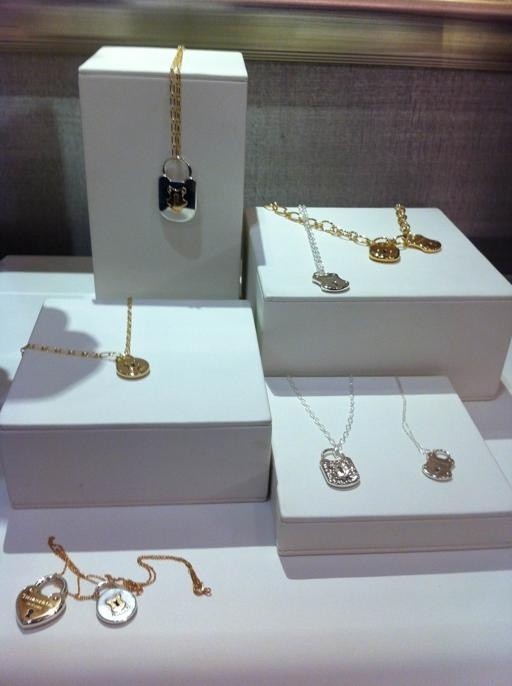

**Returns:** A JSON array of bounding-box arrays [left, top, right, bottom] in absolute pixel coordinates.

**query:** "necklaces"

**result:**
[[157, 42, 198, 225], [286, 372, 359, 489], [263, 194, 444, 292], [20, 295, 149, 380], [395, 376, 456, 483], [13, 535, 211, 631]]
